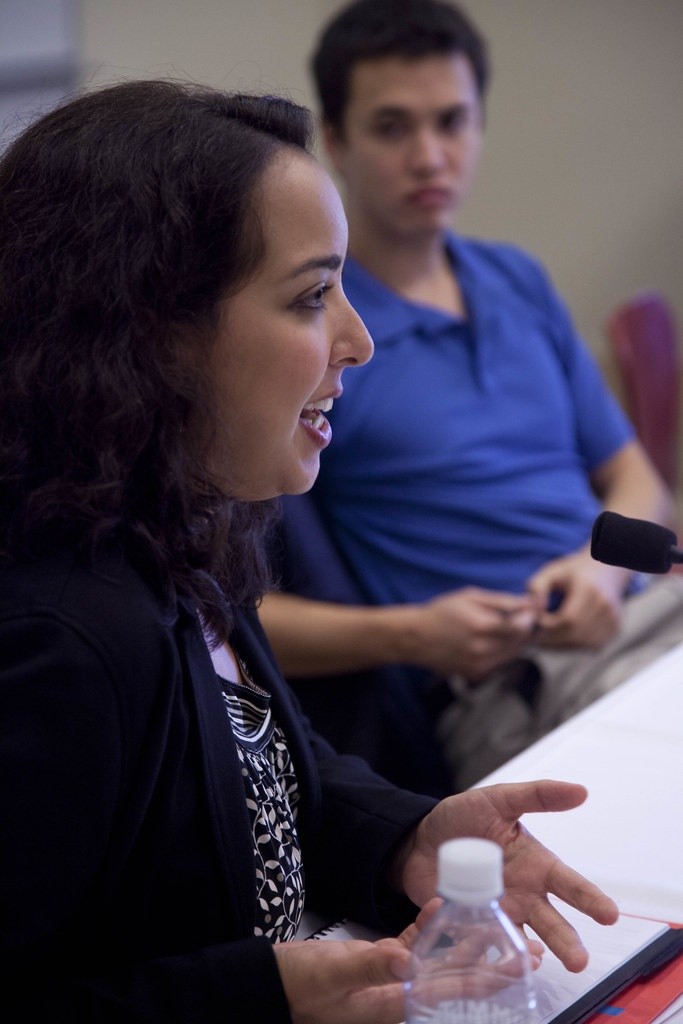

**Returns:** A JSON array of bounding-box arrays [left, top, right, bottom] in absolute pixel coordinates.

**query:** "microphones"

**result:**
[[590, 511, 683, 575]]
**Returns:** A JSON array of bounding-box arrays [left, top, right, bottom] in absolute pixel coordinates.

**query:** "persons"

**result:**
[[2, 82, 620, 1024], [254, 0, 681, 788]]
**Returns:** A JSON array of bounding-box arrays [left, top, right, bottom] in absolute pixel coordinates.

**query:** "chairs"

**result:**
[[609, 292, 683, 495]]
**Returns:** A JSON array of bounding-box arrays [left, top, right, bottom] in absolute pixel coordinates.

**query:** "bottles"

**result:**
[[405, 837, 538, 1024]]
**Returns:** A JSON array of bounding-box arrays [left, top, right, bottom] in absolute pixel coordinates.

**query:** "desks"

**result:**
[[284, 643, 683, 1024]]
